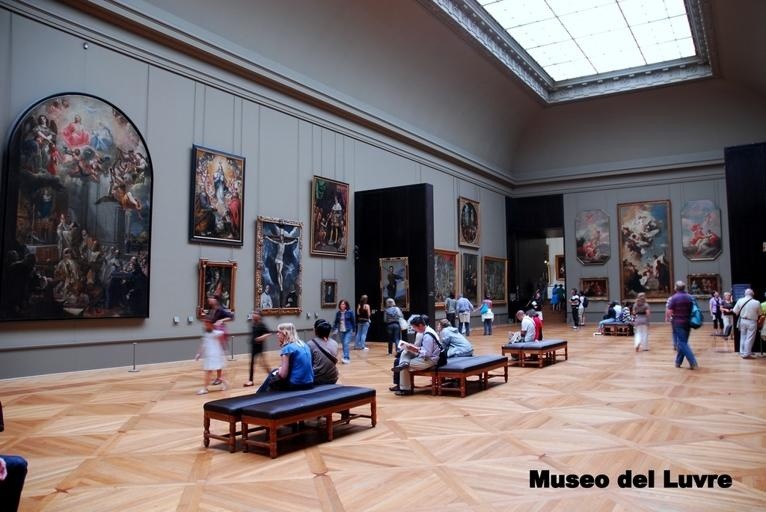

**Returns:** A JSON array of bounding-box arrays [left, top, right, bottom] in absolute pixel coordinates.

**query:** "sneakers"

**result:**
[[362, 347, 369, 350], [573, 326, 578, 329], [197, 390, 209, 396], [354, 347, 361, 350], [594, 332, 601, 334], [213, 378, 223, 385], [483, 333, 485, 335], [488, 333, 492, 335], [466, 333, 469, 335], [342, 359, 351, 364]]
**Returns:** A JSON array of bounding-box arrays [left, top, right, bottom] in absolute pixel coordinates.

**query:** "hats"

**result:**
[[387, 298, 396, 307], [607, 301, 613, 304]]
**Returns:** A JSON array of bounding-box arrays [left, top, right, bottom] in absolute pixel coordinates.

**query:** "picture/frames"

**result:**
[[311, 175, 349, 257], [0, 92, 154, 323], [544, 264, 551, 285], [555, 255, 565, 281], [196, 257, 238, 323], [579, 278, 609, 302], [481, 255, 508, 306], [434, 248, 459, 309], [616, 199, 675, 304], [458, 196, 481, 248], [574, 209, 611, 266], [188, 144, 246, 246], [687, 273, 721, 300], [462, 253, 478, 308], [321, 279, 338, 308], [545, 245, 549, 262], [379, 257, 410, 312], [254, 215, 304, 316], [680, 200, 723, 262]]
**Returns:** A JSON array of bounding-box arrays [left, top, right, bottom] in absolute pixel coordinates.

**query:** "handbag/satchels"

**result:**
[[399, 317, 408, 331], [480, 302, 489, 316], [311, 337, 340, 367], [421, 331, 448, 367], [603, 314, 611, 318], [691, 292, 705, 328], [737, 298, 751, 326]]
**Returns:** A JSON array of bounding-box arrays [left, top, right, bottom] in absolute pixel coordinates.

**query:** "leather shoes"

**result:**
[[395, 390, 412, 396], [691, 366, 698, 368], [391, 361, 410, 372], [243, 383, 254, 387], [676, 363, 681, 367], [390, 385, 400, 392]]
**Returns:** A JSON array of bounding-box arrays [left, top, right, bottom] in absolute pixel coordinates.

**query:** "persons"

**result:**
[[568, 288, 581, 329], [208, 272, 230, 310], [577, 290, 586, 325], [21, 100, 148, 220], [555, 284, 565, 310], [0, 401, 28, 511], [264, 232, 298, 291], [624, 255, 668, 293], [306, 319, 339, 385], [261, 284, 273, 308], [385, 265, 403, 298], [475, 296, 494, 336], [508, 310, 536, 361], [720, 292, 734, 340], [614, 300, 622, 321], [285, 285, 299, 307], [526, 309, 543, 343], [621, 301, 634, 326], [455, 292, 474, 336], [632, 292, 650, 352], [388, 315, 439, 392], [196, 184, 220, 238], [732, 288, 761, 360], [445, 290, 457, 328], [195, 322, 228, 395], [326, 287, 334, 302], [436, 318, 474, 358], [333, 299, 356, 364], [527, 292, 543, 321], [709, 290, 724, 335], [329, 195, 344, 244], [391, 317, 443, 396], [550, 284, 559, 313], [668, 281, 700, 370], [212, 162, 229, 208], [315, 206, 329, 248], [384, 298, 404, 355], [354, 295, 371, 350], [223, 186, 241, 238], [594, 303, 616, 335], [665, 293, 678, 350], [243, 310, 274, 387], [9, 215, 147, 316], [204, 298, 234, 385], [255, 323, 313, 392]]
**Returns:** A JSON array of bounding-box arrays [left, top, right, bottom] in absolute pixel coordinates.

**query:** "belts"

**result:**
[[387, 321, 399, 325], [635, 311, 646, 313]]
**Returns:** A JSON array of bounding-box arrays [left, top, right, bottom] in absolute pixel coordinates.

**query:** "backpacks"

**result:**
[[583, 297, 588, 308], [692, 298, 704, 329]]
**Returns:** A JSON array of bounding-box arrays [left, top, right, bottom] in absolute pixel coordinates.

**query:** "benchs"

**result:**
[[203, 383, 344, 453], [603, 321, 622, 336], [437, 355, 508, 398], [522, 339, 567, 368], [617, 322, 634, 336], [240, 386, 376, 458], [501, 339, 546, 367], [410, 354, 481, 396]]
